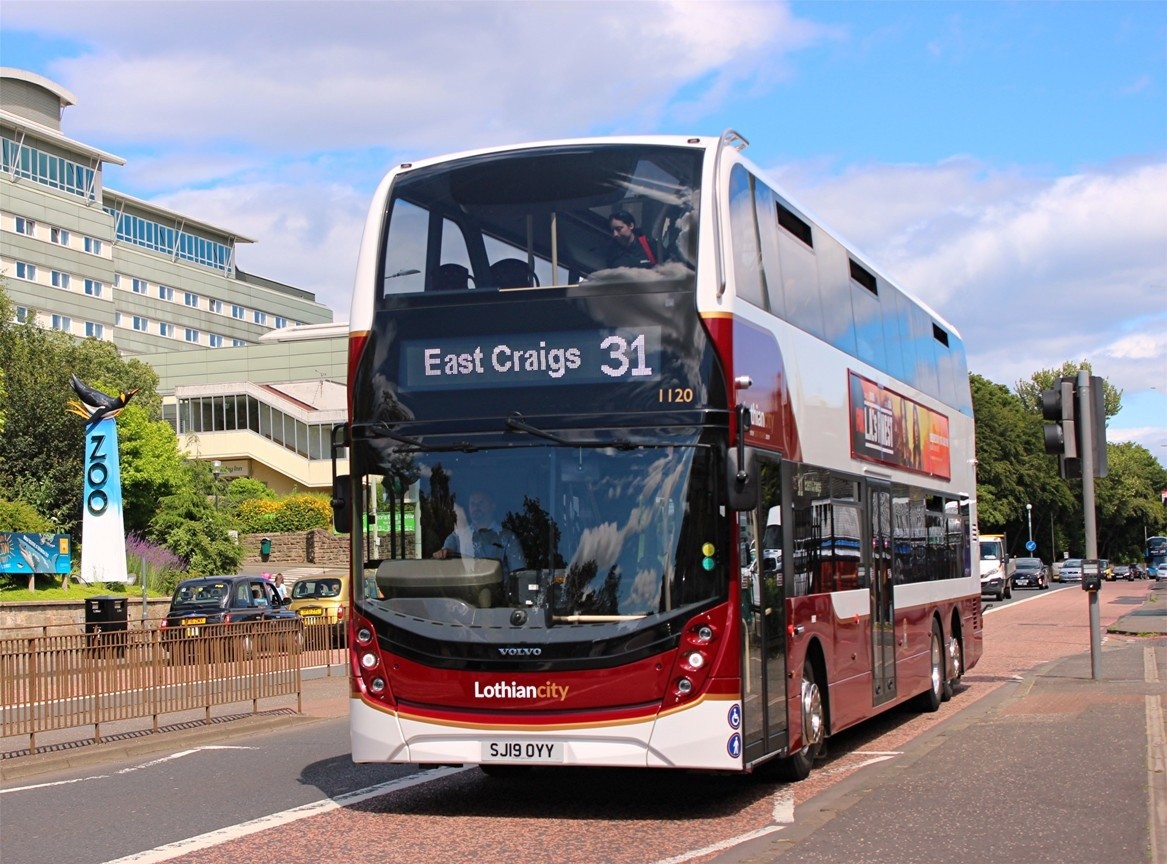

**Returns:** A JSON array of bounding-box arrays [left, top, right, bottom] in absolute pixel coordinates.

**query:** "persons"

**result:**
[[331, 582, 340, 596], [432, 485, 528, 608], [267, 572, 289, 611], [608, 210, 669, 270], [251, 589, 268, 606]]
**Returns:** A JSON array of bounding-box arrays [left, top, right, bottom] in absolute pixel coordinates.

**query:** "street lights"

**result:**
[[1026, 504, 1033, 558]]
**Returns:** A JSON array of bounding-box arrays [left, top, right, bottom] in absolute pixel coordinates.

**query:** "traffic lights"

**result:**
[[1041, 376, 1082, 480]]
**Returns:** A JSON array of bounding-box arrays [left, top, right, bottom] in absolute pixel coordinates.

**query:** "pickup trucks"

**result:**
[[978, 532, 1017, 602]]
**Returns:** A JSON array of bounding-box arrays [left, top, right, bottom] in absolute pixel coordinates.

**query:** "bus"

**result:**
[[1142, 536, 1167, 580], [330, 127, 986, 784]]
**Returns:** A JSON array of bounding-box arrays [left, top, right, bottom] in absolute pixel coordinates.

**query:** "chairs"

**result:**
[[982, 547, 992, 556], [895, 543, 955, 585], [181, 589, 221, 601], [764, 524, 798, 552], [431, 258, 541, 291], [252, 590, 261, 599]]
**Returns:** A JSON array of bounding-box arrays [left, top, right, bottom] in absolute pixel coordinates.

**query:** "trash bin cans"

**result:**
[[261, 538, 271, 554], [84, 595, 128, 658]]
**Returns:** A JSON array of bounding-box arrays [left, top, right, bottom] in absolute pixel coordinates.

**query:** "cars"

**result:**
[[1099, 559, 1145, 579], [1012, 557, 1050, 590], [1058, 558, 1084, 584], [1155, 563, 1167, 581], [1110, 565, 1135, 582], [268, 566, 384, 649], [161, 575, 298, 662]]
